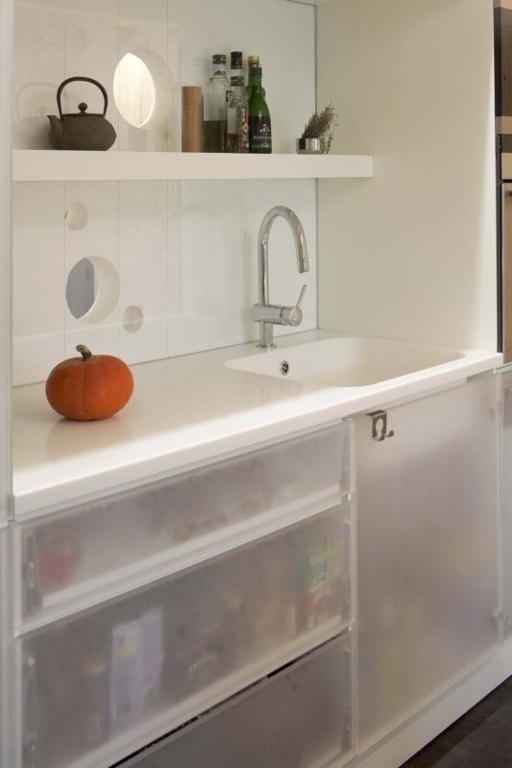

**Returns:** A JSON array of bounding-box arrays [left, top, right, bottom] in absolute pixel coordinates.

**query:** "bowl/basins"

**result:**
[[295, 137, 327, 156]]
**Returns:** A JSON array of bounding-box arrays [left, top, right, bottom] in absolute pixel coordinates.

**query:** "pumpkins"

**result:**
[[45, 345, 134, 420]]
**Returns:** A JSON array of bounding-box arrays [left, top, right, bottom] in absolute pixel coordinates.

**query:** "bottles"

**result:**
[[182, 50, 273, 151]]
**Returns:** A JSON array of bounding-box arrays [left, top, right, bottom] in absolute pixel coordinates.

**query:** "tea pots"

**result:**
[[48, 76, 116, 150]]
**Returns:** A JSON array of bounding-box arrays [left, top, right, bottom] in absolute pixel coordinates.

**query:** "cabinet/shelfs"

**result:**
[[351, 370, 503, 753], [7, 419, 353, 768]]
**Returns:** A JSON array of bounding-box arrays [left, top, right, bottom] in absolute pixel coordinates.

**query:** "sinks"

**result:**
[[222, 335, 470, 388]]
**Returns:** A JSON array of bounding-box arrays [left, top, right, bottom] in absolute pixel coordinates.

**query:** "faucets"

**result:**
[[251, 206, 311, 349]]
